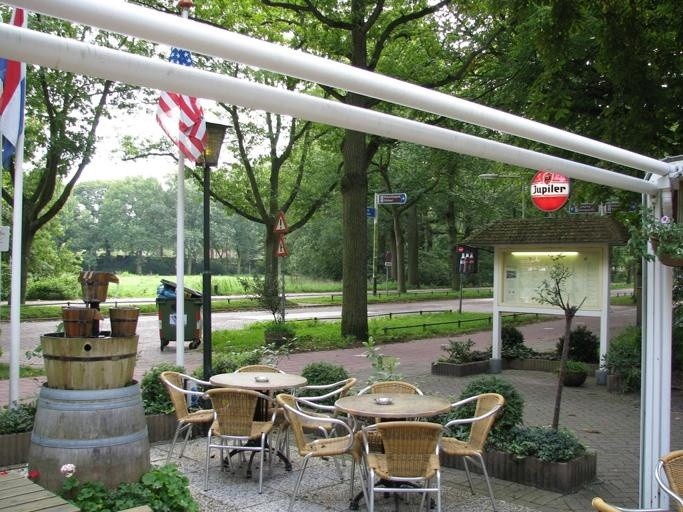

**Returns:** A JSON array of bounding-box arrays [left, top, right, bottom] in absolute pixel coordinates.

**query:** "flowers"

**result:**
[[612, 205, 683, 258]]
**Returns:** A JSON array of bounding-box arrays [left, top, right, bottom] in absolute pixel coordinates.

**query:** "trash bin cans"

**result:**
[[155, 279, 204, 351]]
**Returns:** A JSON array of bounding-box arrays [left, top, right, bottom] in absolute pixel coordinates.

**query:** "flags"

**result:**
[[155, 2, 208, 161], [1, 6, 29, 164]]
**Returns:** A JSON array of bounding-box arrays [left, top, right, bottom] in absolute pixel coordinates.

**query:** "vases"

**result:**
[[650, 235, 682, 268]]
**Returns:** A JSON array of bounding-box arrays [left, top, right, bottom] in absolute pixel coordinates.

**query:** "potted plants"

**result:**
[[237, 273, 295, 348], [556, 362, 588, 387]]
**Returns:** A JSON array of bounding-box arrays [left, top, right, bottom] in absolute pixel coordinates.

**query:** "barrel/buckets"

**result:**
[[78, 270, 119, 305], [38, 330, 140, 392], [60, 302, 95, 338], [27, 379, 152, 500], [108, 301, 139, 337]]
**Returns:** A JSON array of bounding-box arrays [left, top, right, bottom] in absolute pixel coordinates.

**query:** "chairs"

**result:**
[[358, 380, 425, 450], [233, 364, 289, 460], [276, 376, 359, 470], [162, 371, 226, 473], [354, 420, 443, 512], [656, 449, 683, 512], [277, 393, 370, 511], [333, 393, 452, 511], [438, 392, 504, 512]]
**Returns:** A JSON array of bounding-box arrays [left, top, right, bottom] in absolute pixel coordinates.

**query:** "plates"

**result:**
[[373, 398, 392, 405], [254, 376, 269, 382]]
